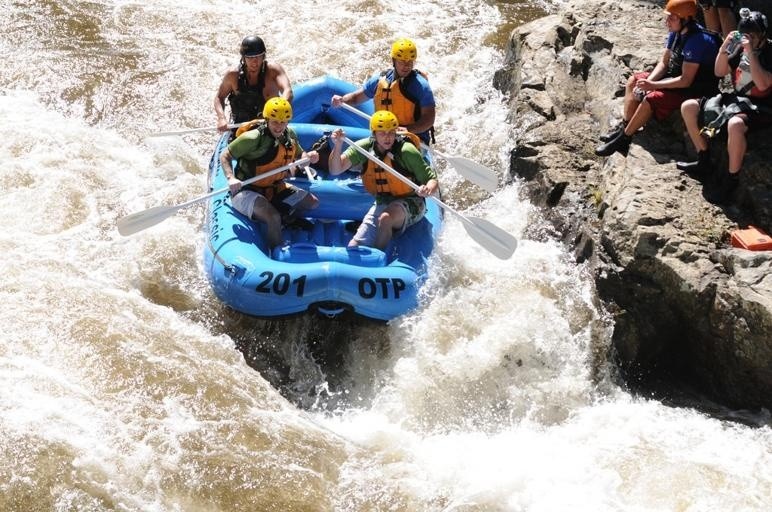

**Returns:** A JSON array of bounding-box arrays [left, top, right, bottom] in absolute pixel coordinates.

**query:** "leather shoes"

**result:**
[[709, 173, 740, 201], [600, 119, 628, 142], [596, 128, 632, 156], [676, 149, 707, 173]]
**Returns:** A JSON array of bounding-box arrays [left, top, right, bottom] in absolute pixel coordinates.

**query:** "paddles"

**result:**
[[334, 128, 517, 260], [143, 121, 251, 147], [117, 156, 311, 236], [339, 101, 498, 192]]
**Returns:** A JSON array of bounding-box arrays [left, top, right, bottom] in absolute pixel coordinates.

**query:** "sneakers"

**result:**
[[289, 218, 315, 230], [346, 220, 362, 233]]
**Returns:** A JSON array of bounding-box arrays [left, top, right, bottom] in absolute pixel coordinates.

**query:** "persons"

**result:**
[[214, 36, 293, 145], [329, 111, 439, 251], [220, 97, 319, 251], [330, 41, 436, 148], [595, 0, 723, 155], [699, 0, 736, 40], [677, 8, 772, 200]]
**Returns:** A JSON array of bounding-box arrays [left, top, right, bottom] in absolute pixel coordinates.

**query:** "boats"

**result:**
[[206, 75, 444, 320]]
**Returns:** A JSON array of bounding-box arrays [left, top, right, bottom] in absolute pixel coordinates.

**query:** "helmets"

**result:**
[[664, 1, 696, 32], [737, 7, 768, 51], [390, 38, 417, 78], [263, 97, 292, 120], [369, 110, 399, 131], [240, 34, 266, 58]]
